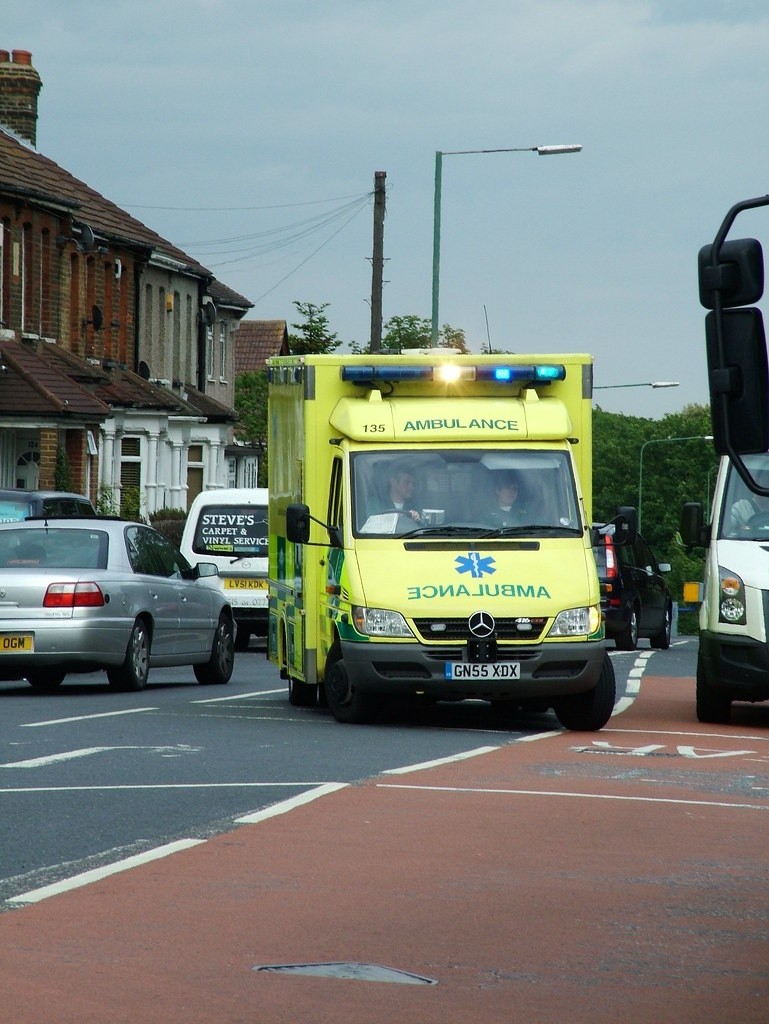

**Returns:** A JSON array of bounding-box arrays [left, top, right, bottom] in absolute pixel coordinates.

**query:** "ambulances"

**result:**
[[266, 343, 616, 732]]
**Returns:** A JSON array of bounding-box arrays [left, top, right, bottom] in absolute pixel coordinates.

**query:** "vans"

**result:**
[[177, 487, 268, 653], [1, 487, 103, 568]]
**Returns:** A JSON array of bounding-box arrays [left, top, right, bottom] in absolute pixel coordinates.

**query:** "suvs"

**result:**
[[590, 522, 672, 652]]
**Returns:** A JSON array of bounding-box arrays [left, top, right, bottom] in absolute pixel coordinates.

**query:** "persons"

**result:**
[[729, 471, 769, 526], [363, 465, 426, 527], [473, 477, 537, 529]]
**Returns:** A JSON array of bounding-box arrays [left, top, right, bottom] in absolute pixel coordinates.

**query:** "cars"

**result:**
[[0, 514, 238, 694]]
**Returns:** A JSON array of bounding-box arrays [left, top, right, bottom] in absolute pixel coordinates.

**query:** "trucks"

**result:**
[[682, 451, 769, 726]]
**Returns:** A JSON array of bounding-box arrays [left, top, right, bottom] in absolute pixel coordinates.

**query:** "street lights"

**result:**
[[637, 435, 713, 530], [429, 144, 583, 345]]
